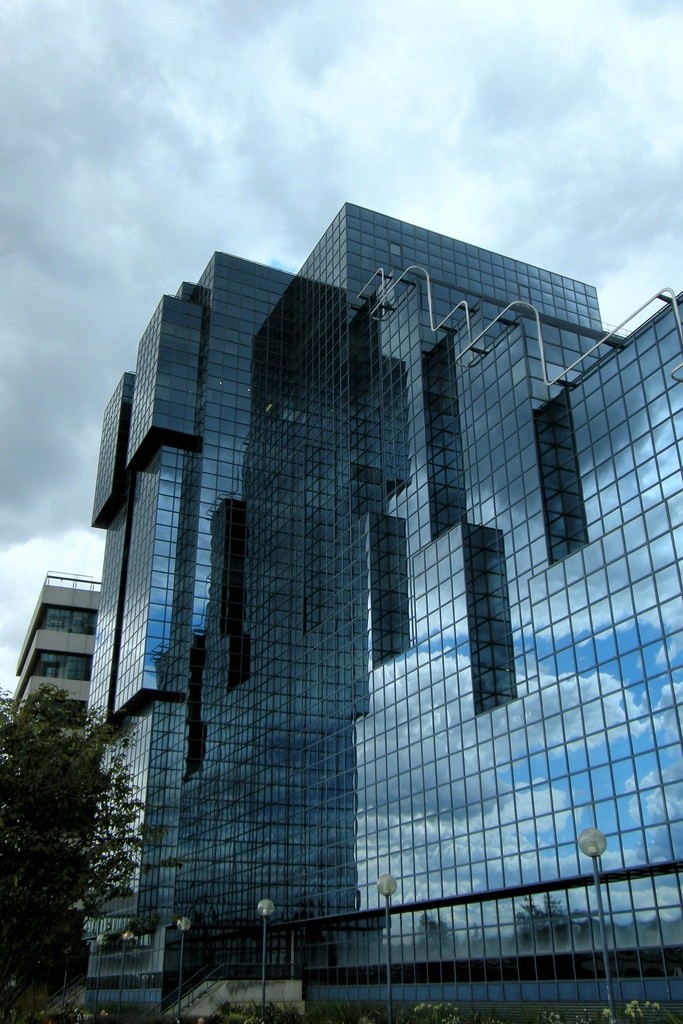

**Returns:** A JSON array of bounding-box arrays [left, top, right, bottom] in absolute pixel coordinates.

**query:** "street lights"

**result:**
[[116, 932, 135, 1024], [256, 898, 275, 1022], [377, 874, 398, 1024], [578, 828, 620, 1024], [176, 917, 192, 1024], [92, 934, 108, 1024]]
